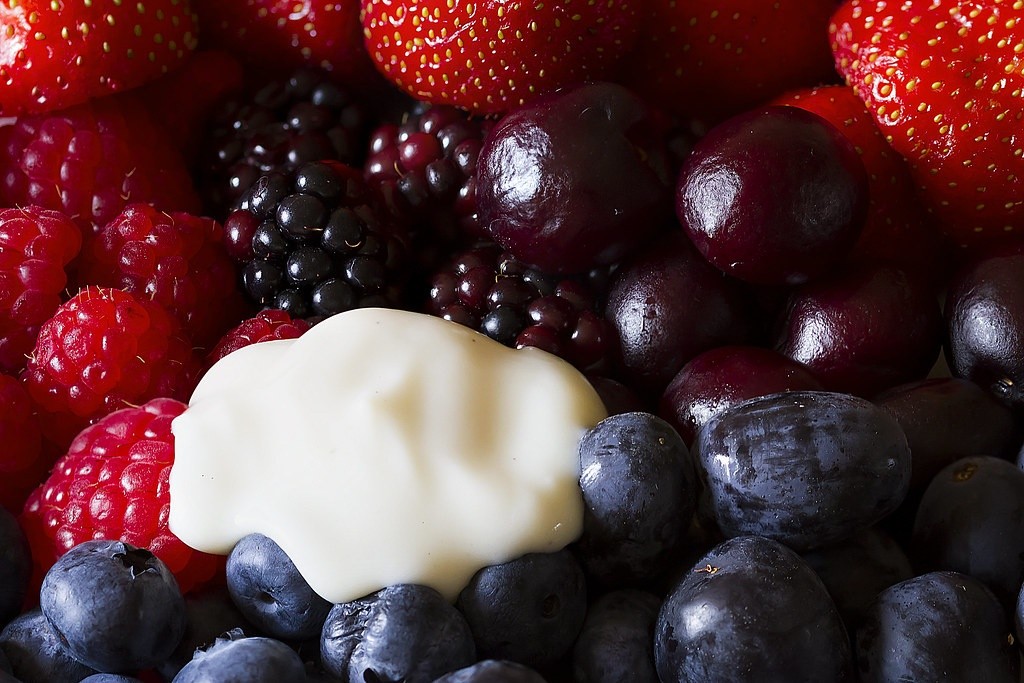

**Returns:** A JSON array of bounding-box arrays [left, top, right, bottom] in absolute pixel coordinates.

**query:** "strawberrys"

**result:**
[[0, 0, 1024, 262]]
